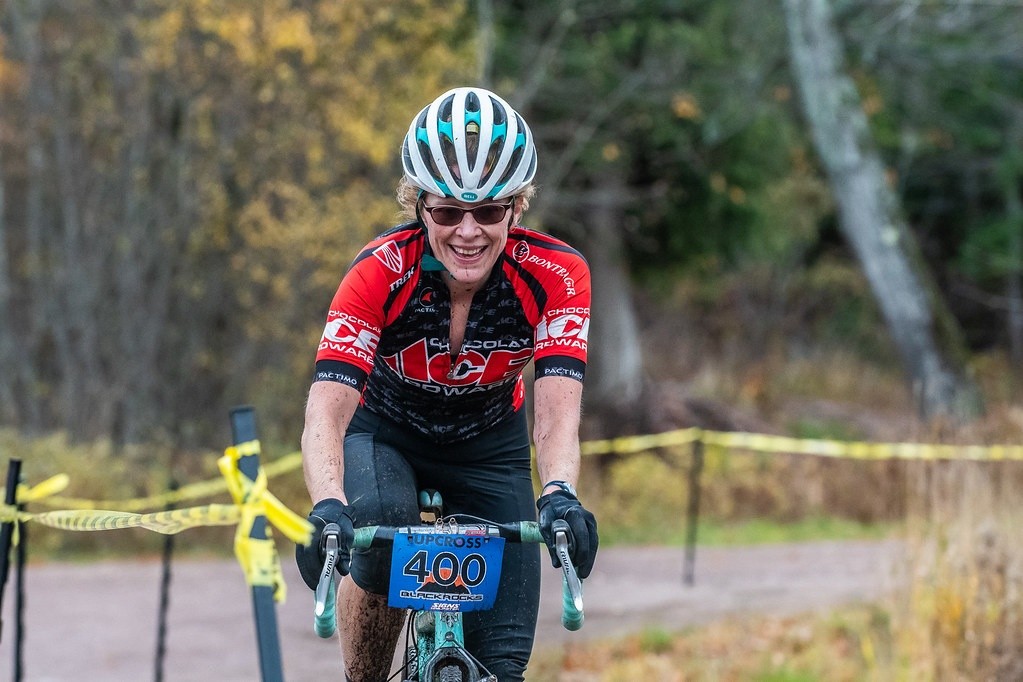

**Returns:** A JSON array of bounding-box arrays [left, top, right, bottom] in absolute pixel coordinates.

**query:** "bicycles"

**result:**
[[315, 488, 587, 682]]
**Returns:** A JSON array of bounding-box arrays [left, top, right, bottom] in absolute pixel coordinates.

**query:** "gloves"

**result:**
[[295, 497, 354, 591], [536, 491, 600, 578]]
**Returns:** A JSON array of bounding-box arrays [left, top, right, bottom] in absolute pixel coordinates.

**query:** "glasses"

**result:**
[[420, 198, 514, 226]]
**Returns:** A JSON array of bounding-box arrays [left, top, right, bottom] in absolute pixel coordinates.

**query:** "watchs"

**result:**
[[538, 481, 577, 498]]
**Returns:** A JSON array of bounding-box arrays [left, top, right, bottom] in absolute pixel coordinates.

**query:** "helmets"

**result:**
[[401, 86, 537, 202]]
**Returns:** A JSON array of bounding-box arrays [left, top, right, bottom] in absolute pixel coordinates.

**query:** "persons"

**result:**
[[296, 87, 600, 682]]
